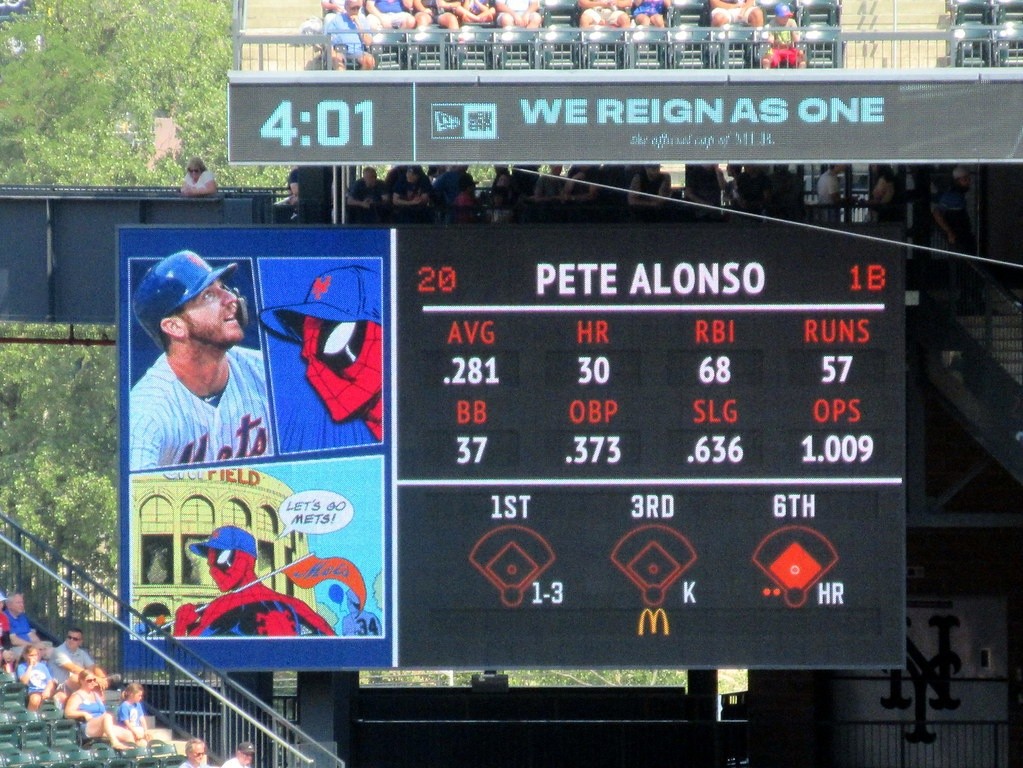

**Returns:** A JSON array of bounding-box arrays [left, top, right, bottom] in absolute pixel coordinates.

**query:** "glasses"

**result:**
[[67, 635, 79, 641], [196, 752, 205, 757], [190, 169, 198, 172], [241, 751, 254, 757], [86, 678, 96, 683]]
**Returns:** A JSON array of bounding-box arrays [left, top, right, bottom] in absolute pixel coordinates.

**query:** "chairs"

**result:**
[[345, 0, 845, 68], [0, 668, 187, 768], [949, 0, 1023, 67]]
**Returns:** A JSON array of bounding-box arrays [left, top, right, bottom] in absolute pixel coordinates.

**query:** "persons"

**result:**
[[16, 645, 67, 712], [115, 682, 153, 747], [181, 158, 218, 196], [413, 0, 461, 29], [46, 628, 121, 706], [709, 0, 764, 28], [861, 164, 895, 223], [763, 4, 808, 69], [321, 0, 366, 30], [178, 738, 210, 768], [932, 164, 974, 258], [319, 0, 376, 70], [634, 0, 671, 28], [345, 163, 802, 224], [816, 161, 845, 223], [456, 0, 496, 24], [288, 165, 300, 204], [366, 0, 416, 28], [3, 593, 55, 670], [221, 742, 255, 768], [129, 250, 273, 470], [0, 592, 24, 673], [65, 670, 136, 750], [496, 0, 542, 28], [579, 0, 632, 27]]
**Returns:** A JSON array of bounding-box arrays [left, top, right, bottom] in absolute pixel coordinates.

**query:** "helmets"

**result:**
[[132, 250, 237, 352]]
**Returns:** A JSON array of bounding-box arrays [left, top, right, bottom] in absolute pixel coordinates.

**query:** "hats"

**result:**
[[239, 742, 254, 752], [952, 167, 977, 180], [345, 0, 361, 9], [0, 593, 8, 601], [452, 173, 480, 190], [774, 3, 794, 17]]
